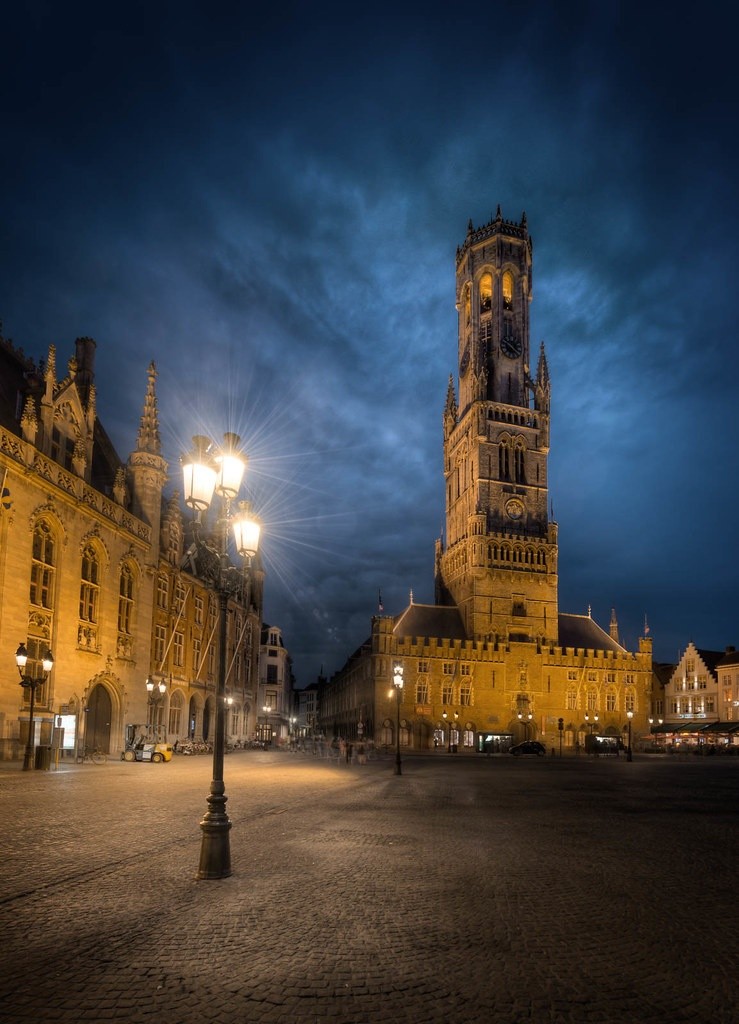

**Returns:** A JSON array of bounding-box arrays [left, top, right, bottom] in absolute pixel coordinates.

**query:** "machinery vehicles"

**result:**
[[120, 722, 173, 762]]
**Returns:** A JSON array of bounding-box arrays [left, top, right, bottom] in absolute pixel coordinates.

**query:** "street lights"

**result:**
[[222, 692, 234, 745], [649, 716, 663, 746], [443, 709, 458, 753], [13, 642, 54, 773], [145, 674, 167, 723], [177, 430, 265, 881], [584, 711, 599, 754], [625, 707, 633, 763], [517, 711, 533, 741], [391, 659, 405, 775]]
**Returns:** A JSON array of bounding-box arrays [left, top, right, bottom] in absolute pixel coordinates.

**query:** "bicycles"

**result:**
[[75, 742, 107, 765], [171, 736, 276, 756]]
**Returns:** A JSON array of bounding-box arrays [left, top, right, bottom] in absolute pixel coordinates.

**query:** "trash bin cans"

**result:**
[[34, 745, 52, 771]]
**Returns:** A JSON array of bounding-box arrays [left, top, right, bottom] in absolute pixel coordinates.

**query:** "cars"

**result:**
[[508, 740, 546, 757]]
